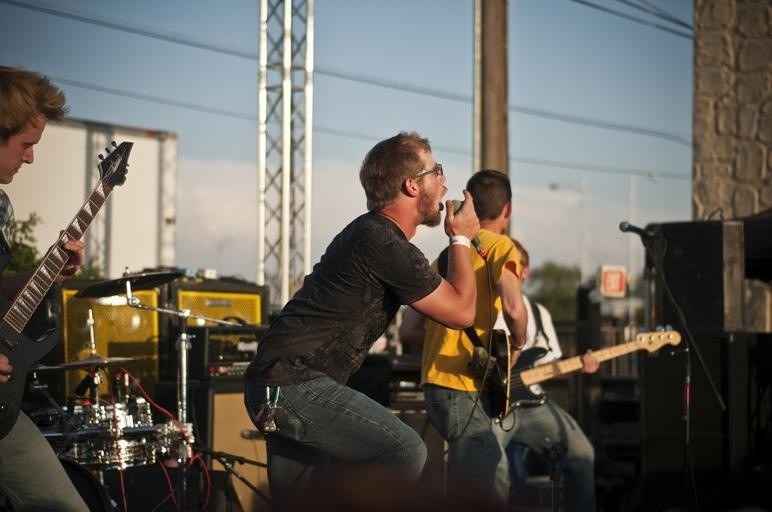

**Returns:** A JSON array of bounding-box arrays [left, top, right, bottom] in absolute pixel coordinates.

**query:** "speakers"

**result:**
[[648, 220, 745, 330], [153, 381, 271, 512], [638, 331, 772, 512]]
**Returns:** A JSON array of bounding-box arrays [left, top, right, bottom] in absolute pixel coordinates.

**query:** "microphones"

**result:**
[[619, 221, 647, 238], [451, 199, 487, 259]]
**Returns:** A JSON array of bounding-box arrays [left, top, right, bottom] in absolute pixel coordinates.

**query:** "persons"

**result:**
[[491, 238, 601, 512], [242, 130, 479, 510], [401, 170, 528, 511], [0, 64, 91, 511]]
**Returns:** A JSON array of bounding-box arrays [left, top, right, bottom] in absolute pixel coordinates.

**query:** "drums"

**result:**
[[28, 407, 67, 433], [56, 396, 156, 471], [155, 421, 194, 459]]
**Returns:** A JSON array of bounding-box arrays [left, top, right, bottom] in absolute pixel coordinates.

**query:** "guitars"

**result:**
[[488, 329, 511, 419], [1, 140, 133, 439], [510, 325, 681, 420]]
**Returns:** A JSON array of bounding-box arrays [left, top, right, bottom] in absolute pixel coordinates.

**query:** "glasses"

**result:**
[[411, 163, 444, 179]]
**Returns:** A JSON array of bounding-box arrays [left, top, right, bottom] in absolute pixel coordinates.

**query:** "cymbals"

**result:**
[[28, 363, 62, 372], [73, 271, 185, 298], [61, 356, 136, 370]]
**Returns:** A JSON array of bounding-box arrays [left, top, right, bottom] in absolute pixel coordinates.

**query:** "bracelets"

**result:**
[[508, 336, 527, 351], [448, 232, 472, 248]]
